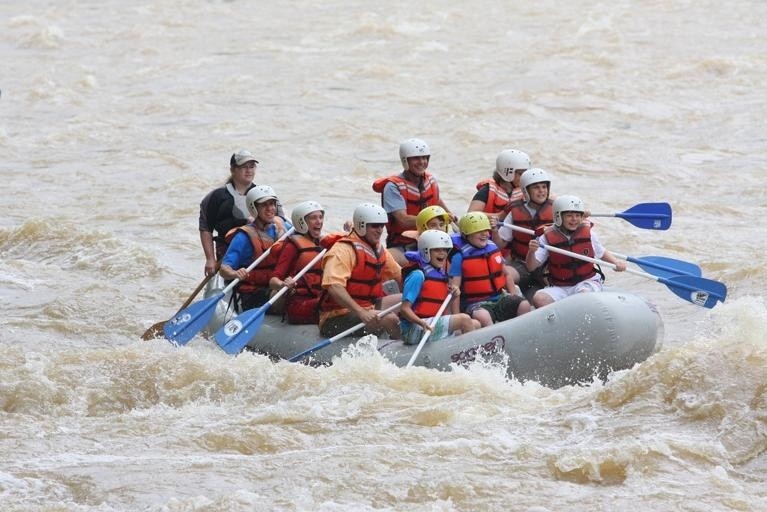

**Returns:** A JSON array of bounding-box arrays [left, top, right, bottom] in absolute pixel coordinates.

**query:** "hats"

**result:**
[[230, 151, 259, 165]]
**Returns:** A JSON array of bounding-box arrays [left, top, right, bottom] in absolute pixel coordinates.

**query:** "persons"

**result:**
[[268, 200, 332, 323], [198, 149, 285, 276], [489, 168, 591, 286], [524, 194, 626, 309], [218, 185, 296, 316], [447, 210, 529, 329], [380, 137, 458, 247], [465, 148, 532, 223], [398, 229, 480, 345], [318, 201, 406, 340], [413, 205, 450, 233]]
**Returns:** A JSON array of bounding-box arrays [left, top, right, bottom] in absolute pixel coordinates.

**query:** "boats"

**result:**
[[200, 263, 665, 391]]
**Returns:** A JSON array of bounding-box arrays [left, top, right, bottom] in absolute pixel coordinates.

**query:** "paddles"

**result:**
[[215, 247, 327, 354], [141, 260, 222, 342], [288, 303, 402, 362], [588, 203, 671, 230], [164, 226, 294, 347], [496, 222, 701, 278], [538, 243, 727, 308]]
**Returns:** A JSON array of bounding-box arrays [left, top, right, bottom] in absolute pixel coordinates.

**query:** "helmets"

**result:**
[[496, 149, 550, 203], [400, 139, 430, 170], [292, 201, 324, 234], [353, 204, 389, 237], [246, 186, 278, 218], [416, 206, 491, 263], [553, 195, 584, 226]]
